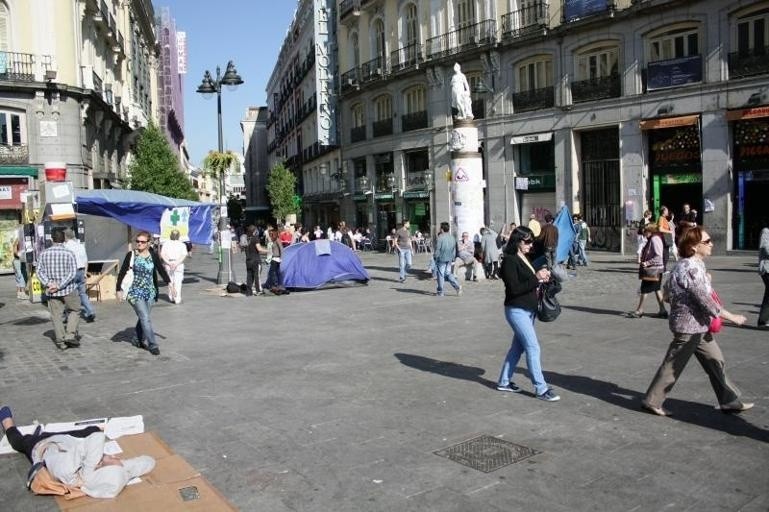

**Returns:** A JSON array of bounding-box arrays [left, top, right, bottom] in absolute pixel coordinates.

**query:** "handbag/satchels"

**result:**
[[119, 249, 136, 302], [536, 271, 561, 322], [639, 253, 661, 281], [708, 290, 723, 333]]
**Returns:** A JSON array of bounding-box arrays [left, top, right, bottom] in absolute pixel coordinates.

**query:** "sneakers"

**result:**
[[435, 291, 445, 296], [32, 424, 42, 436], [472, 277, 480, 282], [454, 274, 458, 280], [456, 284, 463, 296], [534, 387, 561, 402], [494, 273, 500, 280], [496, 382, 523, 393], [19, 293, 30, 300], [488, 275, 494, 279], [65, 339, 80, 348], [0, 406, 12, 431], [59, 343, 68, 349], [16, 292, 19, 298]]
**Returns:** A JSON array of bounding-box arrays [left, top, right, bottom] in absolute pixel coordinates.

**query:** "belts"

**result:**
[[76, 267, 86, 271], [167, 259, 176, 261]]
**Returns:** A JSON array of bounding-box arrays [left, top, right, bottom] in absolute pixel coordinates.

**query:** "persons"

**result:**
[[423, 259, 442, 281], [269, 229, 288, 292], [497, 226, 562, 402], [434, 221, 463, 297], [63, 229, 95, 322], [680, 204, 696, 229], [642, 227, 754, 415], [567, 215, 580, 270], [386, 227, 399, 253], [504, 222, 518, 242], [625, 224, 668, 319], [528, 213, 540, 238], [12, 238, 31, 301], [657, 205, 672, 270], [160, 230, 188, 304], [246, 225, 271, 296], [668, 212, 679, 263], [395, 220, 413, 283], [183, 241, 192, 258], [458, 231, 479, 281], [757, 228, 769, 328], [231, 229, 237, 253], [36, 229, 80, 349], [574, 214, 591, 266], [261, 221, 378, 251], [0, 406, 123, 484], [540, 215, 559, 270], [480, 225, 498, 278], [209, 227, 219, 254], [116, 230, 178, 355], [637, 210, 652, 264], [240, 231, 248, 248]]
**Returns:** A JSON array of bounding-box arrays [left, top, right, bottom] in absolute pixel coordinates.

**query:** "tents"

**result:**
[[268, 239, 370, 290]]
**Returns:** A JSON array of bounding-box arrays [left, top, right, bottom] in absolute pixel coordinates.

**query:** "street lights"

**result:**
[[194, 59, 242, 284]]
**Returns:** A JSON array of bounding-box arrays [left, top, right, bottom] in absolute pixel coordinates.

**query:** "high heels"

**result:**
[[651, 310, 669, 320], [722, 400, 754, 414], [640, 397, 673, 417], [757, 318, 767, 328], [629, 309, 646, 318]]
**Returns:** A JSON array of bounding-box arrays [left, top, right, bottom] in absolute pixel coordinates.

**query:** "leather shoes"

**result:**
[[132, 335, 147, 348], [150, 348, 160, 356], [86, 314, 95, 323], [169, 300, 175, 304], [63, 318, 68, 324]]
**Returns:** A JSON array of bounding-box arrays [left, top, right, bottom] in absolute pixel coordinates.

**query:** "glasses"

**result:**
[[462, 235, 468, 238], [572, 219, 579, 220], [690, 238, 711, 246], [135, 239, 150, 245], [521, 236, 537, 245]]
